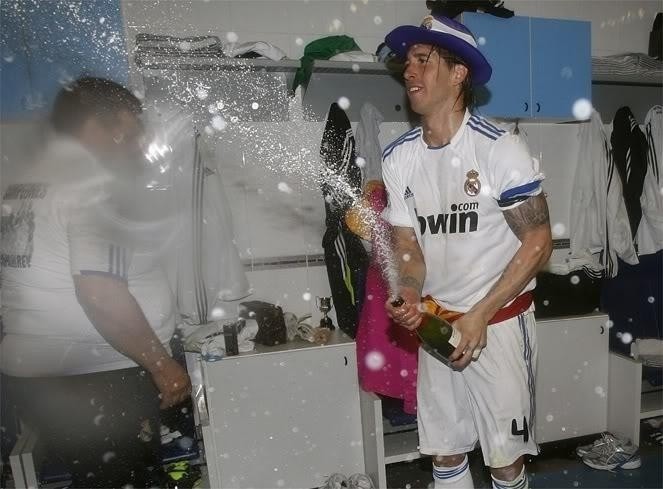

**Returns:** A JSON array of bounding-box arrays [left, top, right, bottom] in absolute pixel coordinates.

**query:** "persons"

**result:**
[[0, 76, 192, 489], [376, 15, 553, 488]]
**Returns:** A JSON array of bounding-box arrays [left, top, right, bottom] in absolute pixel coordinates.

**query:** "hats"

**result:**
[[385, 14, 492, 86]]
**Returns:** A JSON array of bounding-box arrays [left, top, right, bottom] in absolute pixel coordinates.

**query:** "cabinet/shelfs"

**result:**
[[530, 311, 645, 447], [183, 328, 389, 489], [453, 12, 593, 121]]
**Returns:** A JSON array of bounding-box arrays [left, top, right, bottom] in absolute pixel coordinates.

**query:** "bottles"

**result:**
[[390, 296, 483, 365]]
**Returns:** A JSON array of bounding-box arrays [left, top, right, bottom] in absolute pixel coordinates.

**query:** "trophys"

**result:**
[[315, 294, 336, 330]]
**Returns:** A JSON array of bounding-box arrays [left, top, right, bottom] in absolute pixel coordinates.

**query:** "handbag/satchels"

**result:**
[[238, 300, 286, 346]]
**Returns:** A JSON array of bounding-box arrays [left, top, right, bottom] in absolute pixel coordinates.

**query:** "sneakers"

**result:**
[[576, 433, 631, 457], [582, 441, 642, 473]]
